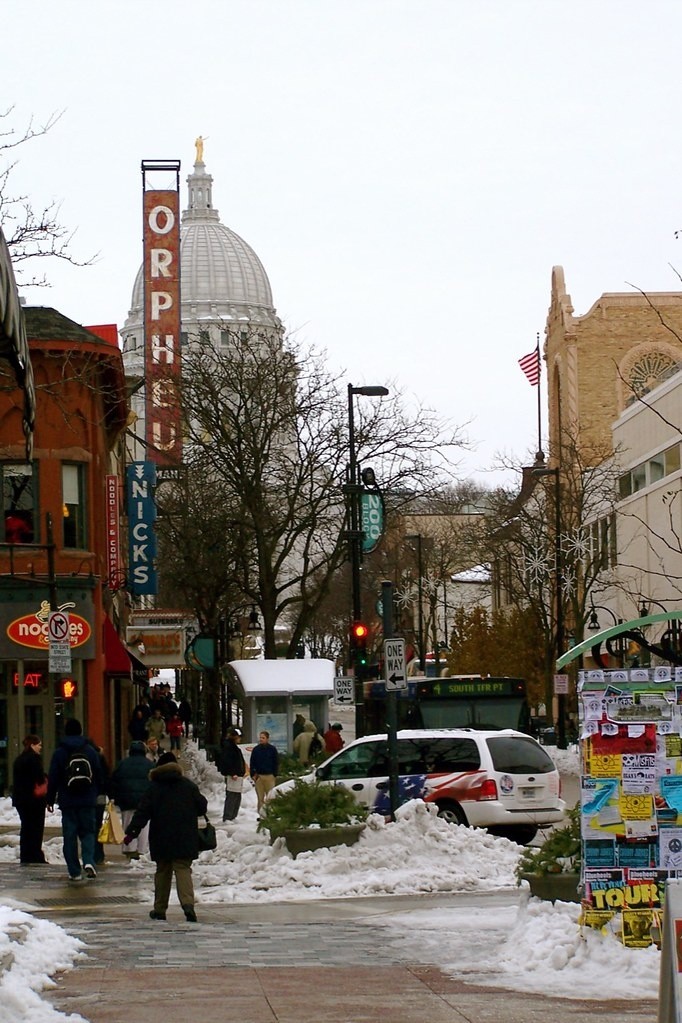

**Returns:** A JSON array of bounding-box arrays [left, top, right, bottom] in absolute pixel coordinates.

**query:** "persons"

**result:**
[[124, 752, 208, 923], [106, 741, 155, 860], [132, 681, 193, 765], [293, 713, 345, 769], [46, 718, 111, 880], [219, 724, 247, 826], [249, 731, 281, 814], [5, 509, 31, 544], [11, 734, 49, 866]]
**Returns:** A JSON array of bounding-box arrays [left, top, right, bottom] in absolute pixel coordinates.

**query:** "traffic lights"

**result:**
[[352, 623, 369, 680], [58, 681, 79, 700]]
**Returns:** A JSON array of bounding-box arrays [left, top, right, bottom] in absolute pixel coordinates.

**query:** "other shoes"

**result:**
[[183, 904, 197, 922], [149, 910, 167, 920]]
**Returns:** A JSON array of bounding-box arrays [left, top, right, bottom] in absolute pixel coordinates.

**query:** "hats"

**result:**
[[156, 751, 178, 765], [22, 734, 41, 749]]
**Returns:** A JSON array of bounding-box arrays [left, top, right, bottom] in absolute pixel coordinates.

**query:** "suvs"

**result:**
[[265, 728, 567, 843]]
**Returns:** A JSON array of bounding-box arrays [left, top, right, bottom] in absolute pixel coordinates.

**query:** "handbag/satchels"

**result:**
[[33, 776, 48, 797], [198, 814, 217, 851], [98, 801, 125, 845]]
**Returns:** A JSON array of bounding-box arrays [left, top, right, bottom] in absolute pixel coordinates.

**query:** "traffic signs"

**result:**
[[384, 639, 407, 691]]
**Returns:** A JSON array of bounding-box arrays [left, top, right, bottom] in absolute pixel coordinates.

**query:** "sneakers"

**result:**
[[68, 875, 82, 880], [84, 864, 97, 878]]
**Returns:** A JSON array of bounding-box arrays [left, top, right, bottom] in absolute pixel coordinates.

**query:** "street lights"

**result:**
[[638, 601, 672, 666], [588, 604, 623, 667], [348, 386, 393, 733], [405, 535, 425, 662], [531, 470, 567, 750]]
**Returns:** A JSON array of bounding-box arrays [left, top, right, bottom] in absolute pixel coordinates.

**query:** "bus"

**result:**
[[352, 676, 530, 763], [409, 660, 447, 676]]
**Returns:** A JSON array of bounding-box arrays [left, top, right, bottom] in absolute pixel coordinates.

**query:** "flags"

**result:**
[[518, 344, 541, 386]]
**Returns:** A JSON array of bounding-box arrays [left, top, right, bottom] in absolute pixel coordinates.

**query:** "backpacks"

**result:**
[[64, 742, 93, 795], [307, 731, 322, 762]]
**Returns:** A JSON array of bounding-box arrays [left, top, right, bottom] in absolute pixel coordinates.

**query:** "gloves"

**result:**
[[124, 835, 133, 846]]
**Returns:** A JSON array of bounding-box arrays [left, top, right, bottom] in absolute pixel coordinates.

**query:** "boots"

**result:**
[[176, 749, 181, 759]]
